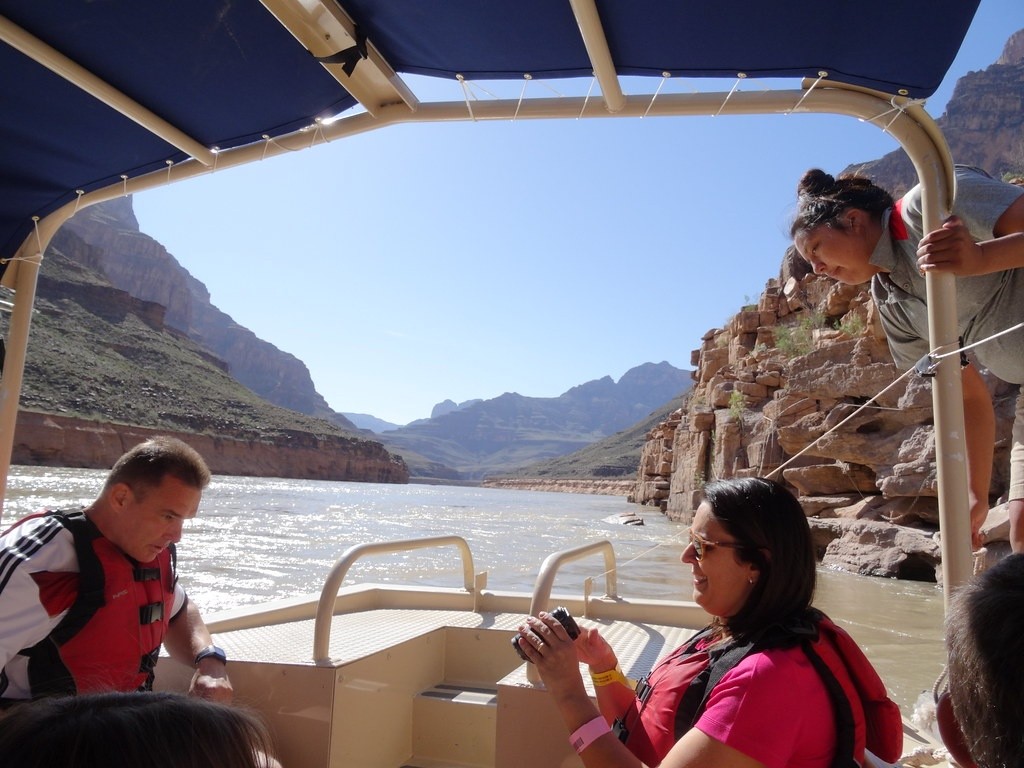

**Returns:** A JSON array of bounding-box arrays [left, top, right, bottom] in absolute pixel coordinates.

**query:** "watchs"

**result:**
[[195, 645, 226, 666]]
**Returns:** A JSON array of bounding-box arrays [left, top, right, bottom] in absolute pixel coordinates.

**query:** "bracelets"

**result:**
[[568, 715, 612, 755], [588, 659, 638, 691]]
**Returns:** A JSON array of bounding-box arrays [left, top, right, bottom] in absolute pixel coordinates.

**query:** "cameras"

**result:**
[[511, 606, 581, 664]]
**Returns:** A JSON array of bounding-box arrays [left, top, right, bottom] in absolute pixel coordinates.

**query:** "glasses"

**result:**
[[688, 527, 745, 561]]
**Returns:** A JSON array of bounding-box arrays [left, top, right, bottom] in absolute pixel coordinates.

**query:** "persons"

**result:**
[[0, 435, 234, 707], [789, 164, 1024, 553], [936, 553, 1024, 768], [517, 476, 867, 768]]
